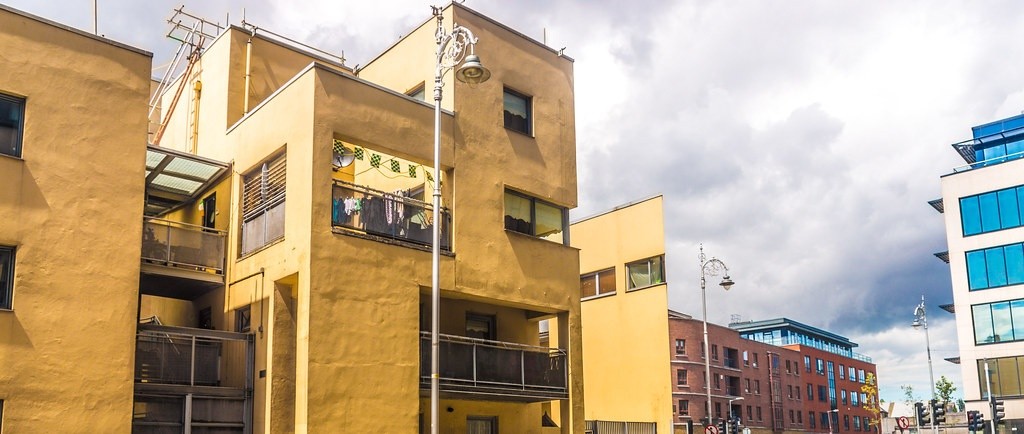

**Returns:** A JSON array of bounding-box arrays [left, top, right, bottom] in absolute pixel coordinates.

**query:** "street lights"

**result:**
[[431, 6, 492, 434], [911, 295, 935, 399], [827, 409, 840, 434], [730, 397, 744, 418], [698, 242, 734, 425]]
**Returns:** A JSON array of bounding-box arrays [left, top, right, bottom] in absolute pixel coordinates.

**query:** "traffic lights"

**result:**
[[967, 411, 984, 431], [731, 419, 738, 433], [932, 399, 946, 426], [991, 397, 1004, 429], [915, 402, 931, 426]]
